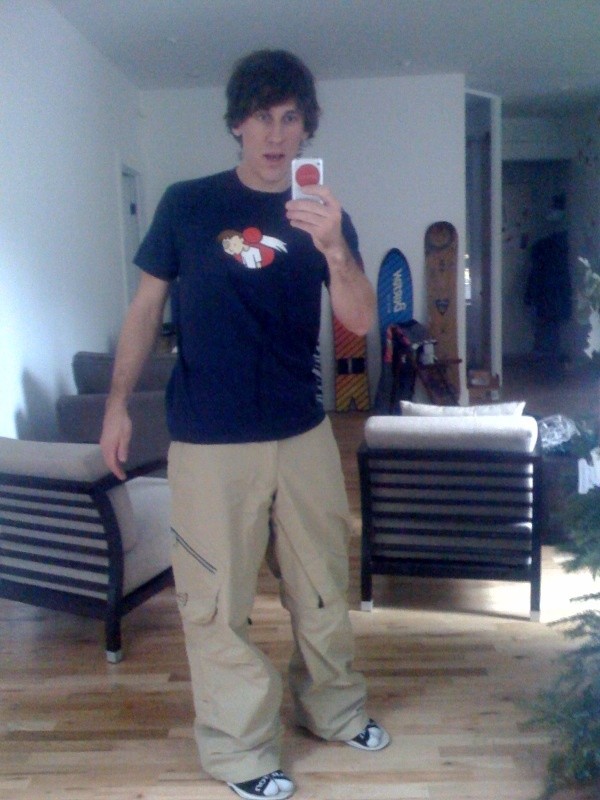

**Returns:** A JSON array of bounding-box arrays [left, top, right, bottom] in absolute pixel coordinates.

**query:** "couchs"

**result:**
[[57, 352, 179, 458]]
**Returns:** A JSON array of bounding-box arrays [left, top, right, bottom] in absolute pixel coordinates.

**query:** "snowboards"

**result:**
[[423, 220, 459, 401], [377, 247, 414, 403], [328, 261, 372, 412]]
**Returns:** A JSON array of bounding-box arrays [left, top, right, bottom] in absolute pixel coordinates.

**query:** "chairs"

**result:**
[[0, 435, 176, 662], [353, 413, 554, 625]]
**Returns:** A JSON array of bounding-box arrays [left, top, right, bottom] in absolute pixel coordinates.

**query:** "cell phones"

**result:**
[[291, 158, 324, 205]]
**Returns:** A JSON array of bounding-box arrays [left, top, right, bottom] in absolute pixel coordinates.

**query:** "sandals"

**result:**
[[228, 770, 294, 800], [345, 718, 391, 751]]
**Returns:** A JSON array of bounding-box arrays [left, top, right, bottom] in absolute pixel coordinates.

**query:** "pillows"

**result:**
[[400, 399, 525, 416]]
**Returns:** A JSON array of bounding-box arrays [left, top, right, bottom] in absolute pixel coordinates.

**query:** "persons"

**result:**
[[101, 46, 390, 800]]
